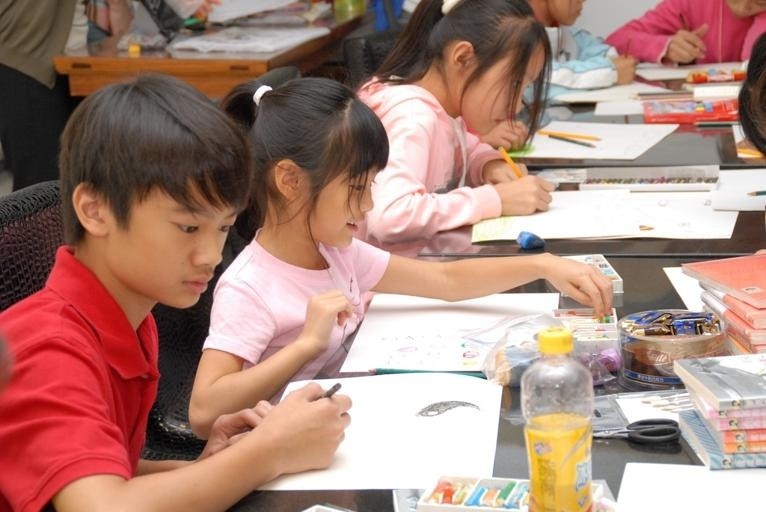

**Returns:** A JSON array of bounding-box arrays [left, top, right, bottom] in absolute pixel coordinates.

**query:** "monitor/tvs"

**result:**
[[673, 352, 766, 471], [699, 283, 766, 329], [700, 305, 766, 352], [700, 294, 766, 346], [681, 254, 766, 309]]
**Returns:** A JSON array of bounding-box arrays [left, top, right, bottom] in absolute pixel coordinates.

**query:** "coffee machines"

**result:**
[[593, 419, 681, 443]]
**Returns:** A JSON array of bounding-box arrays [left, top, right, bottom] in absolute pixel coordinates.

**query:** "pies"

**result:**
[[429, 481, 530, 508], [586, 177, 718, 184]]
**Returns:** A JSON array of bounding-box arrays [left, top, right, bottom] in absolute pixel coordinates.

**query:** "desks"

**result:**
[[53, 13, 369, 108], [223, 60, 766, 512]]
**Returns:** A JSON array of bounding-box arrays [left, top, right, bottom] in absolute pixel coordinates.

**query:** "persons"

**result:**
[[85, 1, 225, 48], [187, 77, 616, 449], [0, 1, 132, 189], [517, 1, 638, 104], [467, 117, 531, 153], [350, 0, 558, 261], [0, 68, 353, 509], [603, 1, 766, 65]]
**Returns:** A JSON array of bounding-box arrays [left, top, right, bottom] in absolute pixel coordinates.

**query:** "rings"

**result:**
[[498, 146, 524, 178], [624, 38, 631, 58], [537, 129, 601, 148], [318, 383, 341, 400], [680, 14, 700, 53], [368, 368, 488, 379], [747, 191, 766, 196], [693, 121, 742, 127]]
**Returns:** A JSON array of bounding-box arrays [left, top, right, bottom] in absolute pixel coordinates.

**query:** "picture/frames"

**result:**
[[53, 13, 369, 108]]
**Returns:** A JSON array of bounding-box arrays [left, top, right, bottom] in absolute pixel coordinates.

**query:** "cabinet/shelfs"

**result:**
[[521, 327, 596, 512]]
